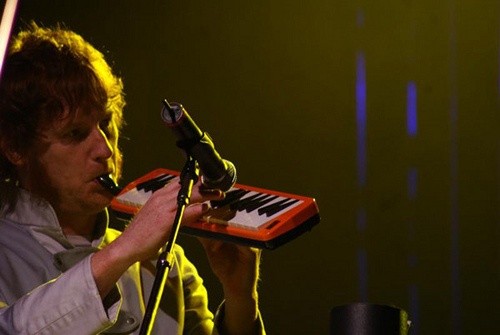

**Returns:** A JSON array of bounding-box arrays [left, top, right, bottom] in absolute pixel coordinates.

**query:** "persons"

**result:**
[[0, 22, 265, 335]]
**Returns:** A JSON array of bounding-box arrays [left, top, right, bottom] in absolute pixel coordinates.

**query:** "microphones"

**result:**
[[161, 99, 237, 192]]
